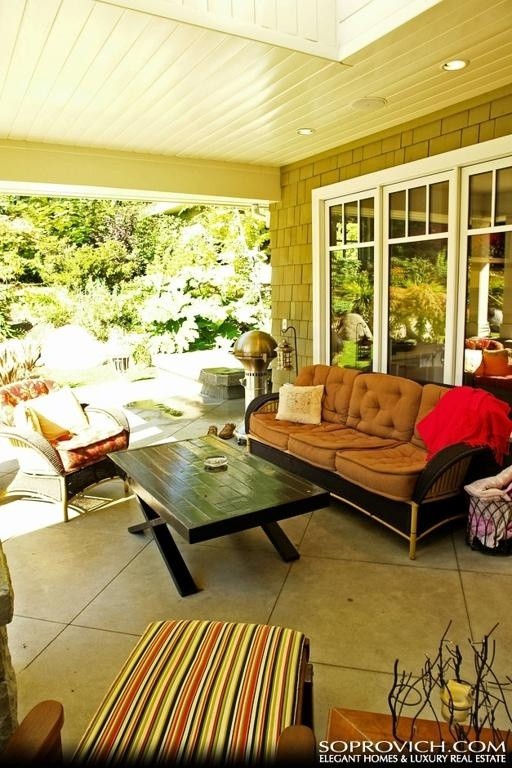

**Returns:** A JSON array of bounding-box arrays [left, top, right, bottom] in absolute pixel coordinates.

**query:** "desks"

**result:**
[[326, 706, 512, 768]]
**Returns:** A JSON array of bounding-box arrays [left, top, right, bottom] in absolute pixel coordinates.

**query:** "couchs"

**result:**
[[245, 363, 511, 559]]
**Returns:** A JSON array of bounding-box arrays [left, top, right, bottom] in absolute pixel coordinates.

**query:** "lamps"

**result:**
[[273, 325, 299, 379]]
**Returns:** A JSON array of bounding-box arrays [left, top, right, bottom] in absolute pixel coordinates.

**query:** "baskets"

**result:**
[[468, 495, 511, 556]]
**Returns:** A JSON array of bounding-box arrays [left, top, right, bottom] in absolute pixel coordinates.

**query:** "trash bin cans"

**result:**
[[112, 357, 130, 373]]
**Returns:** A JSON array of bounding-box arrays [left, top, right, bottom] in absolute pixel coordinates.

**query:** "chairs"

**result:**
[[0, 617, 322, 768], [0, 401, 130, 525]]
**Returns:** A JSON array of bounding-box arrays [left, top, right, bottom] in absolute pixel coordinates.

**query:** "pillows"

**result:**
[[275, 382, 325, 425], [26, 385, 89, 440]]
